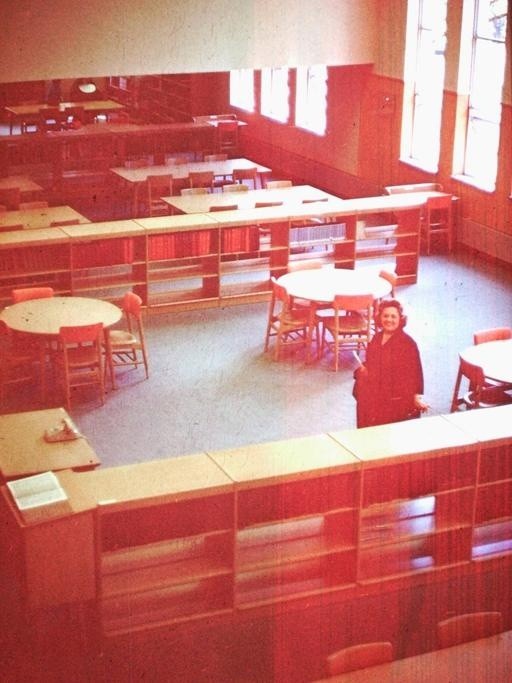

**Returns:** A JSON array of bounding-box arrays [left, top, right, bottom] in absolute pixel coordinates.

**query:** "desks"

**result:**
[[378, 181, 461, 255]]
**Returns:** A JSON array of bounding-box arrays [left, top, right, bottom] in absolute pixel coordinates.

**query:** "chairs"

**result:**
[[448, 326, 511, 413], [0, 286, 150, 411], [323, 611, 505, 676], [4, 92, 141, 132], [263, 267, 398, 374]]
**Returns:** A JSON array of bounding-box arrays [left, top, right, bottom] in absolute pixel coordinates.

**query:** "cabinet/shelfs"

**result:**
[[1, 122, 247, 189], [0, 405, 510, 652], [0, 192, 429, 318]]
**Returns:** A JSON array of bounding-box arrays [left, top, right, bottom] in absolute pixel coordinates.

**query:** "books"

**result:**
[[7, 469, 69, 523]]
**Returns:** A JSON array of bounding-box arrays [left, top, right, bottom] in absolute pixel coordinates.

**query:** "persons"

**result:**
[[351, 298, 431, 429]]
